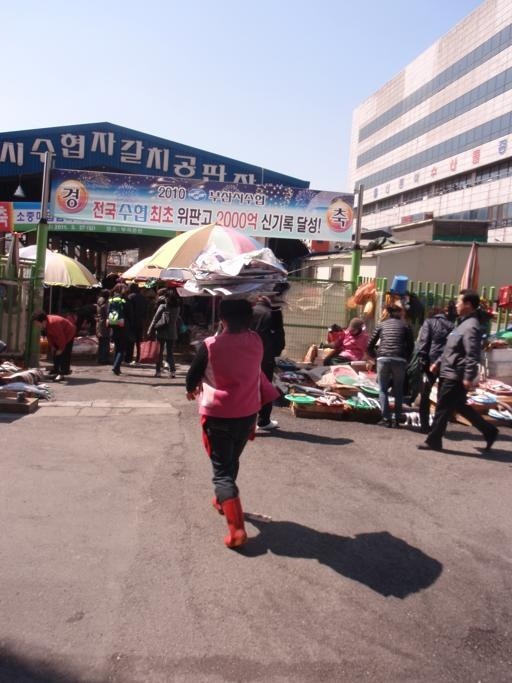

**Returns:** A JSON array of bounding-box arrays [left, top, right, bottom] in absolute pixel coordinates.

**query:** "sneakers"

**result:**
[[153, 372, 161, 378], [392, 421, 399, 428], [256, 420, 279, 430], [169, 371, 175, 379], [377, 420, 392, 427]]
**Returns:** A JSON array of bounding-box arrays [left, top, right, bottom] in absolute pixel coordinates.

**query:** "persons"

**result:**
[[30, 309, 77, 382], [185, 296, 282, 548], [318, 317, 373, 367], [414, 299, 459, 432], [246, 295, 287, 431], [365, 303, 415, 429], [94, 280, 188, 379], [417, 289, 499, 450]]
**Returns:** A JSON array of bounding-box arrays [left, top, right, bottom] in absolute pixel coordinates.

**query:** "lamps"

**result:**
[[14, 173, 27, 200]]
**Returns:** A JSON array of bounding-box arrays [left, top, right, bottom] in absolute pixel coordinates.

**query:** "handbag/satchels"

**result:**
[[153, 311, 170, 331]]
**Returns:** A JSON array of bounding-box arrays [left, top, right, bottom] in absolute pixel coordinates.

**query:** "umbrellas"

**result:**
[[0, 244, 52, 260], [458, 242, 482, 293], [17, 251, 100, 290], [118, 217, 287, 295]]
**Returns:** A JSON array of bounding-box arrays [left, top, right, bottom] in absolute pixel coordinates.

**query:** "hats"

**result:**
[[347, 318, 364, 332], [327, 324, 341, 332]]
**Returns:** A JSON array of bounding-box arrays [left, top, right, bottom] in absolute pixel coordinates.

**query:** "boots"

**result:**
[[212, 495, 225, 514], [221, 497, 248, 549]]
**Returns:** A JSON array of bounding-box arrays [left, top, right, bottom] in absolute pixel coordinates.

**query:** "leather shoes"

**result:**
[[54, 377, 64, 381], [416, 442, 440, 450], [486, 428, 499, 449]]
